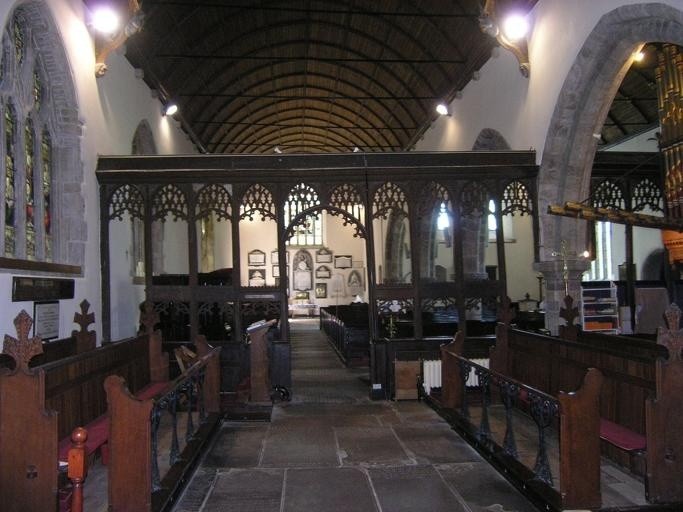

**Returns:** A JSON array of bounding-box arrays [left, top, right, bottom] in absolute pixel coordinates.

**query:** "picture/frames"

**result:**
[[247, 249, 365, 299]]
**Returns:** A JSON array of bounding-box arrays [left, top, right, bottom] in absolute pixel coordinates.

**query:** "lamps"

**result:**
[[83, 2, 145, 79], [162, 101, 178, 117], [478, 2, 530, 77]]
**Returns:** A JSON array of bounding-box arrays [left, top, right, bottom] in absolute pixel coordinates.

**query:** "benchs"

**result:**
[[319, 302, 495, 369], [441, 301, 682, 512], [0, 299, 222, 511]]
